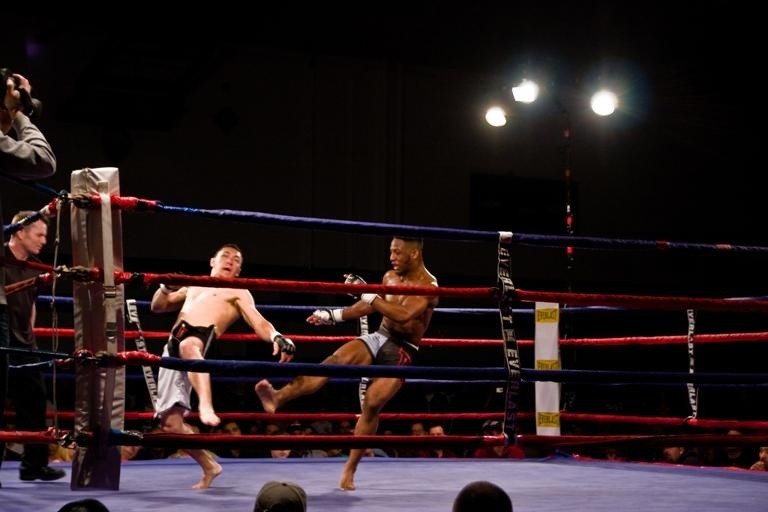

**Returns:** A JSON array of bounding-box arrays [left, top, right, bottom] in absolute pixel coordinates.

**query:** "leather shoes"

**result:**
[[19, 465, 64, 481]]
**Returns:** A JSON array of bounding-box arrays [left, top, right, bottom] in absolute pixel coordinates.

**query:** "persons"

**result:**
[[254, 229, 439, 492], [1, 209, 66, 481], [0, 66, 56, 182], [452, 480, 513, 512], [149, 243, 296, 489], [1, 376, 766, 475]]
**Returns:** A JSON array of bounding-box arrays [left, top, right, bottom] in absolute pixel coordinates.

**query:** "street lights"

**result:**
[[486, 65, 628, 288]]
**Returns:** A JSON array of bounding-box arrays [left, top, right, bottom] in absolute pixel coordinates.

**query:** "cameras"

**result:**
[[0, 68, 43, 120]]
